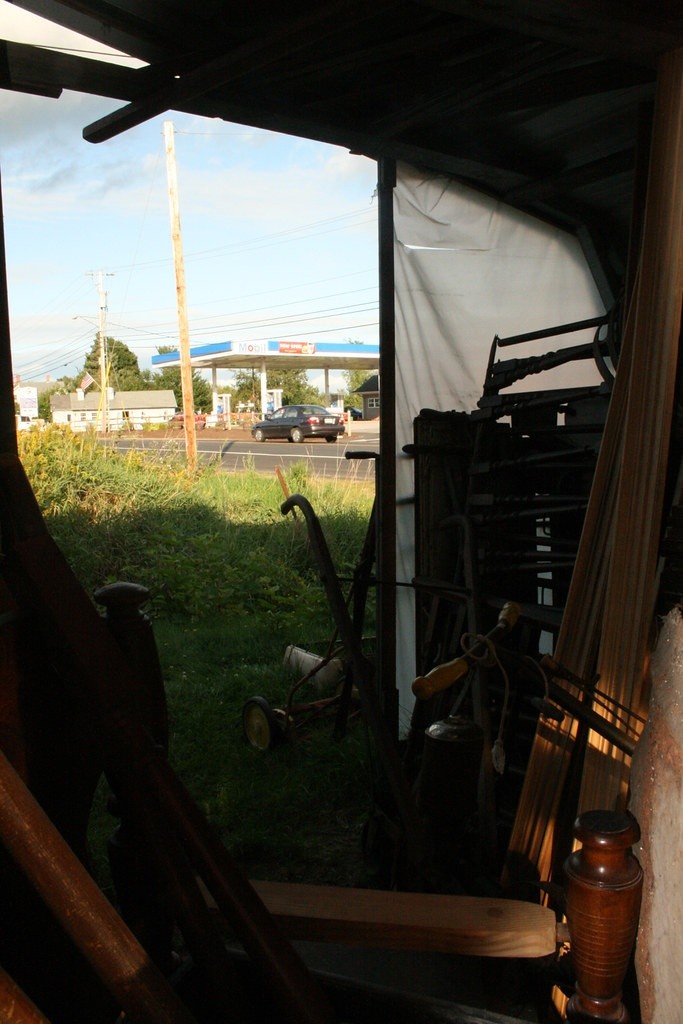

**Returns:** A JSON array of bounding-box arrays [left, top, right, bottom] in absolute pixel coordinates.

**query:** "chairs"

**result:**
[[0, 443, 642, 1023]]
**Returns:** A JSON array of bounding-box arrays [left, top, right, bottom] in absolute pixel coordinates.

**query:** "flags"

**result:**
[[81, 373, 94, 391]]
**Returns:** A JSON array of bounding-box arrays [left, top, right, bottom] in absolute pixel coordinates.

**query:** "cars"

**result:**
[[248, 405, 347, 444]]
